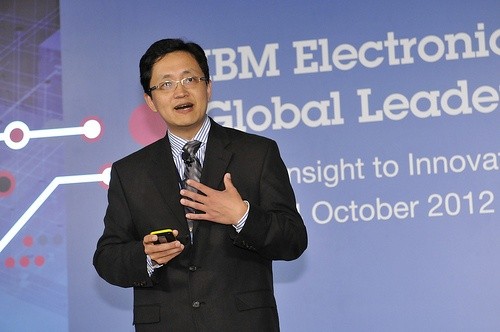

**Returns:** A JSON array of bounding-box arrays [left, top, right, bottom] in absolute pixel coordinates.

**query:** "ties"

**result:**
[[182, 140, 203, 244]]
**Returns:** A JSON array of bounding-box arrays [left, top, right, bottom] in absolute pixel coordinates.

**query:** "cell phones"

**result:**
[[151, 229, 176, 245]]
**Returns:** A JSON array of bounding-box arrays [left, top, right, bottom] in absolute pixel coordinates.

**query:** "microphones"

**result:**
[[184, 158, 192, 164]]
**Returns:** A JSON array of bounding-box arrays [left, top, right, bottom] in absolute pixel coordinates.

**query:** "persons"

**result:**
[[92, 38, 309, 332]]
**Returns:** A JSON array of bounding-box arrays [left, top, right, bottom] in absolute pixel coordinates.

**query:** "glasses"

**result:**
[[145, 77, 208, 93]]
